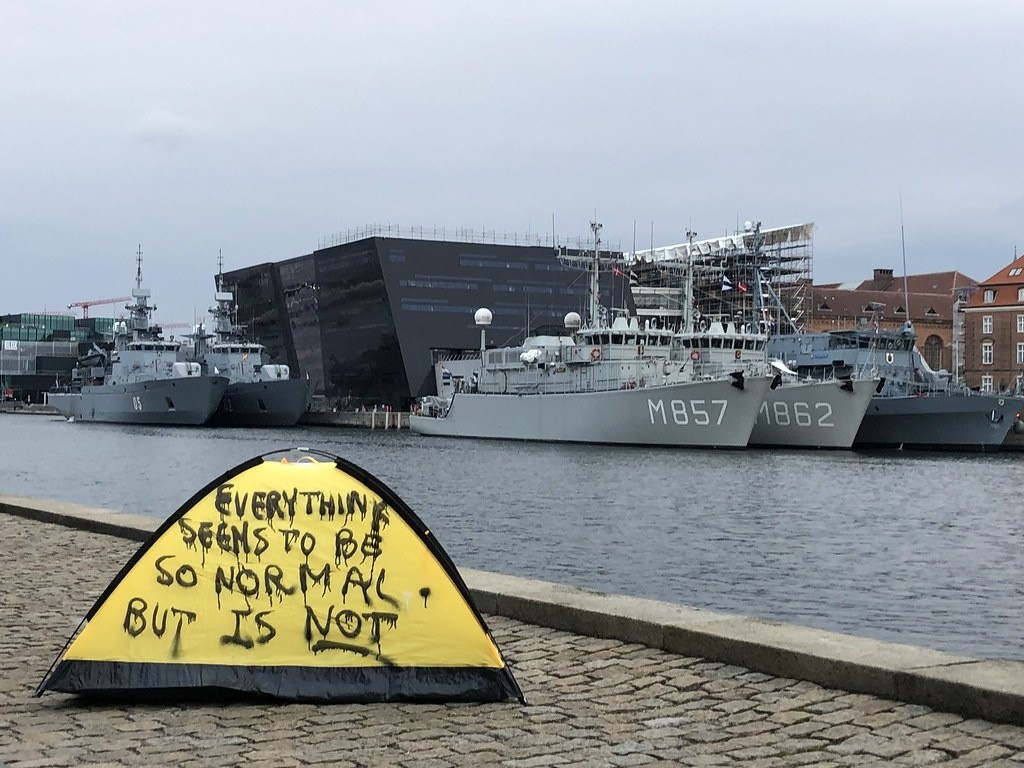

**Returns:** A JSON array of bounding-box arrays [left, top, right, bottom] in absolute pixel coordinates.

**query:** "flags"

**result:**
[[874, 314, 879, 334], [629, 270, 639, 286], [721, 275, 732, 292], [442, 367, 451, 386], [738, 282, 747, 293], [613, 264, 622, 277]]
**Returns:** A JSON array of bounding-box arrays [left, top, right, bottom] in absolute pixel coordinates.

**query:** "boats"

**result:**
[[409, 207, 781, 452], [650, 218, 886, 452], [201, 247, 319, 429], [751, 222, 1024, 454], [47, 244, 231, 427]]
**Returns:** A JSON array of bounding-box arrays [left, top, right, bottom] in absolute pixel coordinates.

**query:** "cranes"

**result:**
[[159, 323, 192, 329], [67, 298, 131, 319], [29, 311, 76, 316]]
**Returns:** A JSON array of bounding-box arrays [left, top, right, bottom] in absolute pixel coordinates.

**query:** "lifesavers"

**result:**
[[638, 344, 644, 355], [591, 348, 600, 358], [690, 350, 700, 361], [735, 350, 741, 359]]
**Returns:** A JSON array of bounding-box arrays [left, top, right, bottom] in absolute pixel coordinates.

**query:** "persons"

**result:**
[[412, 406, 424, 416], [970, 384, 981, 394], [386, 405, 393, 413], [619, 381, 637, 390], [361, 404, 369, 412], [1000, 378, 1009, 393], [26, 395, 32, 406], [948, 375, 954, 389], [914, 367, 922, 392]]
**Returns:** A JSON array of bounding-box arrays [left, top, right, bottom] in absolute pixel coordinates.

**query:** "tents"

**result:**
[[35, 446, 528, 706]]
